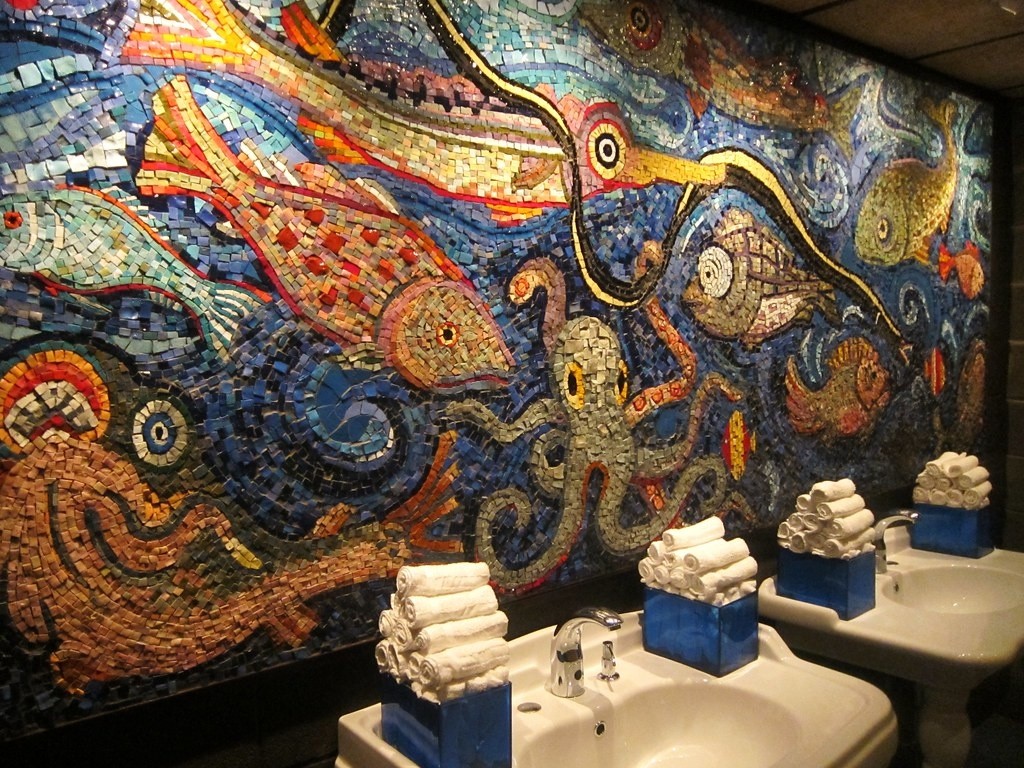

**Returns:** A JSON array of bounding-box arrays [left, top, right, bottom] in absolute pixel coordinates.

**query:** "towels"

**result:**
[[776, 477, 876, 561], [911, 450, 993, 510], [638, 516, 758, 606], [373, 561, 510, 703]]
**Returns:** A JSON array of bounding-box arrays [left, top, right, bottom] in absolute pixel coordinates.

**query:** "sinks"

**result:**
[[515, 682, 801, 768], [883, 563, 1024, 616]]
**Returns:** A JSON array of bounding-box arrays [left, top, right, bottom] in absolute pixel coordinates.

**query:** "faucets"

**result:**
[[549, 605, 625, 698], [873, 508, 921, 574]]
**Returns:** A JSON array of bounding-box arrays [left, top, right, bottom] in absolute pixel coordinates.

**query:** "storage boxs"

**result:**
[[777, 547, 876, 622], [642, 585, 758, 678], [911, 503, 995, 559], [379, 672, 513, 768]]
